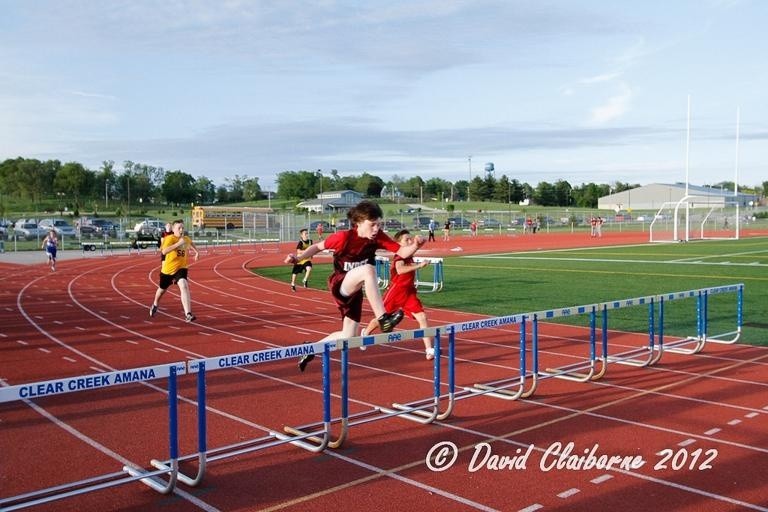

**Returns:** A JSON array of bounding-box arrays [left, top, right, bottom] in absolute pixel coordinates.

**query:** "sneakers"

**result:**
[[425, 347, 443, 360], [377, 306, 404, 333], [183, 312, 196, 323], [302, 279, 307, 289], [149, 303, 159, 317], [359, 327, 367, 351], [297, 339, 315, 372], [47, 259, 56, 272], [290, 283, 296, 293]]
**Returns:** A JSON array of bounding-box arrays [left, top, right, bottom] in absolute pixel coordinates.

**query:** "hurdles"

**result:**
[[81, 237, 279, 256], [374, 255, 443, 293], [1, 284, 744, 512]]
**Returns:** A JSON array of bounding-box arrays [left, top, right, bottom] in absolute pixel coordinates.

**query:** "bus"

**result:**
[[190, 205, 280, 231]]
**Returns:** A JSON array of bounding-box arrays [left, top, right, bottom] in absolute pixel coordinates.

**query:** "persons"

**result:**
[[156, 222, 174, 269], [315, 222, 323, 242], [590, 217, 597, 238], [596, 217, 604, 238], [282, 198, 428, 373], [536, 215, 541, 231], [148, 219, 200, 324], [289, 227, 313, 293], [720, 215, 730, 232], [469, 218, 478, 238], [532, 219, 538, 234], [427, 218, 435, 242], [360, 228, 443, 362], [41, 229, 59, 272], [526, 216, 533, 233], [441, 219, 451, 243]]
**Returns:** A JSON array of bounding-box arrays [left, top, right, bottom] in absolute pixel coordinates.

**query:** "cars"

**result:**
[[307, 215, 585, 230], [14, 217, 167, 240]]
